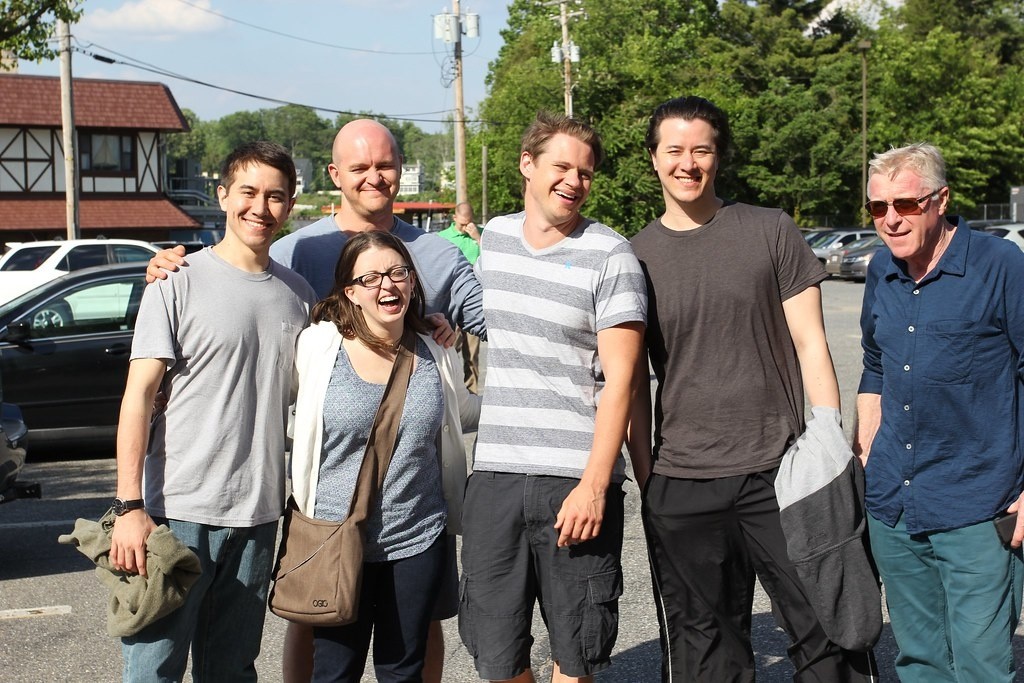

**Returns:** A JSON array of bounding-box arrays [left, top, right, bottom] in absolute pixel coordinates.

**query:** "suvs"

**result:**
[[0, 239, 168, 336], [968, 218, 1024, 253]]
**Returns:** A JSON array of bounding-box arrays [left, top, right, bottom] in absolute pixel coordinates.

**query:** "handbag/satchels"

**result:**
[[269, 494, 374, 626]]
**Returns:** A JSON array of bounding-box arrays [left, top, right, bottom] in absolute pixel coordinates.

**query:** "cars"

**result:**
[[798, 225, 887, 284], [0, 260, 164, 449]]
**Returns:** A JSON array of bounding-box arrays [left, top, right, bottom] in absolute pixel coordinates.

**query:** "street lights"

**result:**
[[855, 41, 872, 230]]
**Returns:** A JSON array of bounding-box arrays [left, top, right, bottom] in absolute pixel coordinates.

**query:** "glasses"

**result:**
[[865, 186, 946, 218], [349, 266, 412, 288]]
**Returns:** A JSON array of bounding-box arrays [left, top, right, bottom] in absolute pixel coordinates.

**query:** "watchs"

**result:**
[[111, 497, 144, 516]]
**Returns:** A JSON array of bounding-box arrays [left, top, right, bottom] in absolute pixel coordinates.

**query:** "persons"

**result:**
[[625, 91, 881, 683], [453, 116, 652, 683], [439, 200, 483, 396], [143, 115, 491, 683], [107, 141, 458, 683], [151, 228, 642, 683], [849, 139, 1024, 683]]
